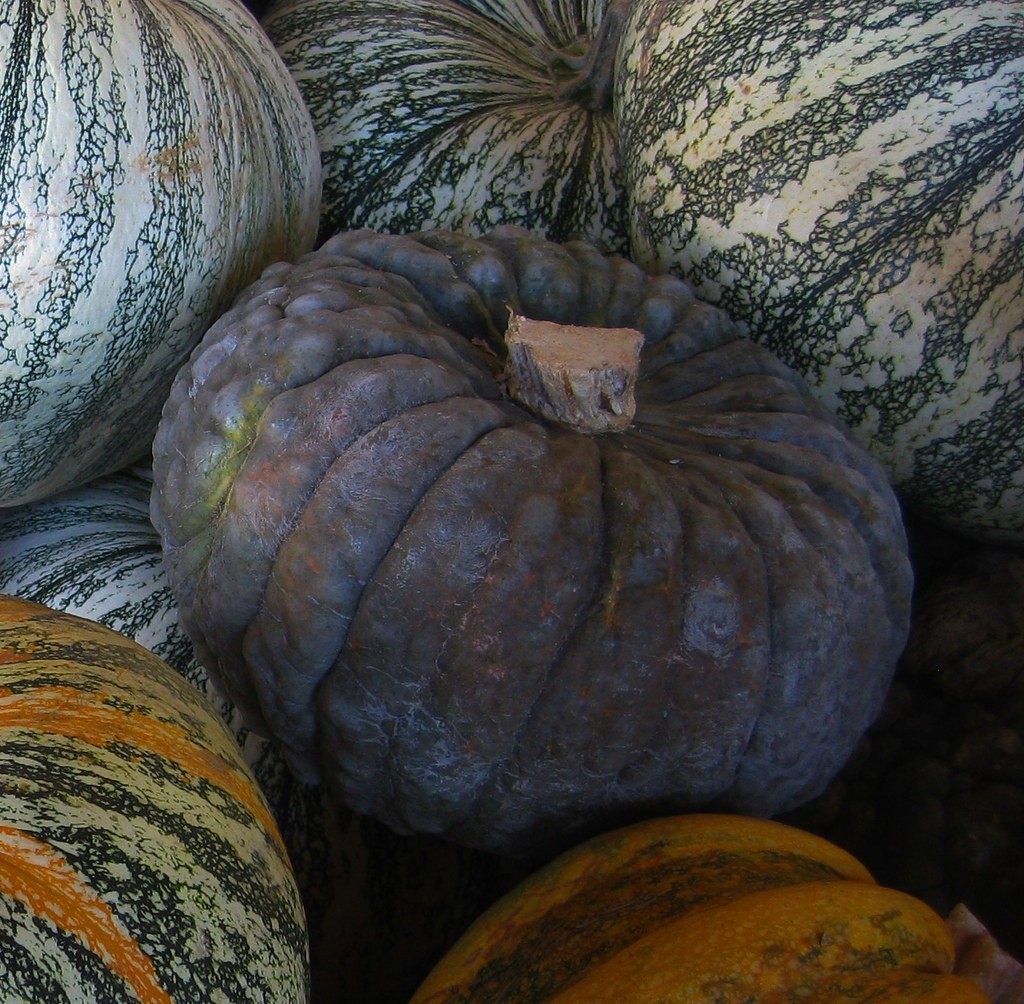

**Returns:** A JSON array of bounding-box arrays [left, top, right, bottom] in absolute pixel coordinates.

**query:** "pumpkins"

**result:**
[[0, 0, 1024, 1004]]
